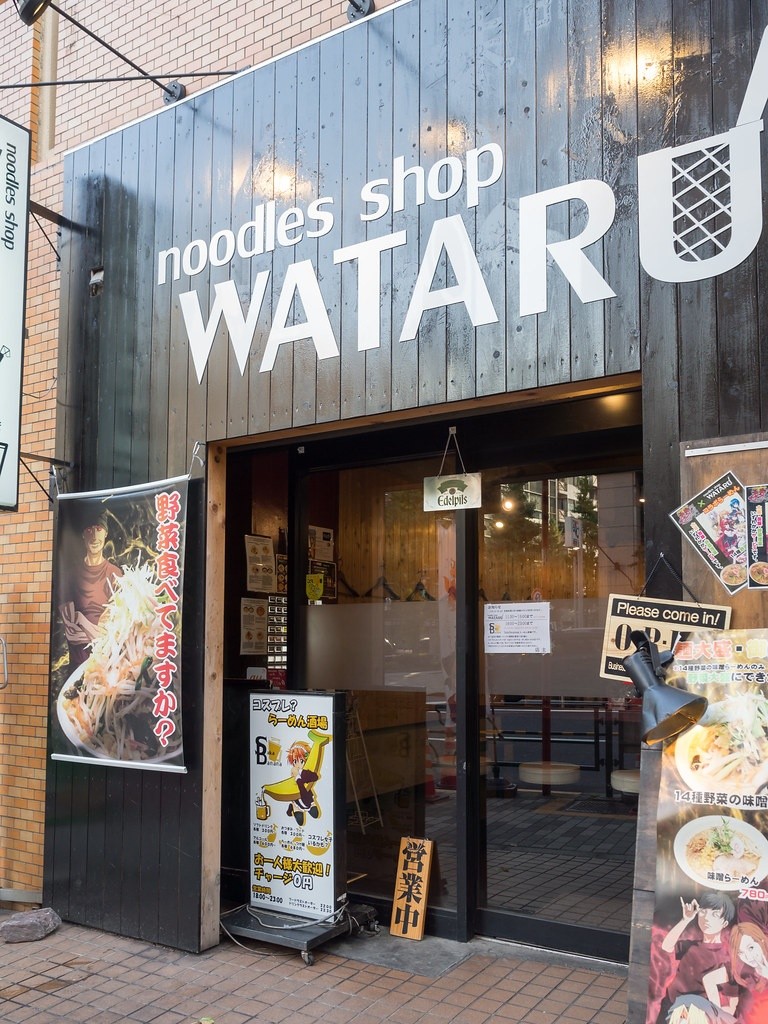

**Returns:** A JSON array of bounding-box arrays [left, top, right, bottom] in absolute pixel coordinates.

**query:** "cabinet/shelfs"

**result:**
[[340, 688, 426, 876]]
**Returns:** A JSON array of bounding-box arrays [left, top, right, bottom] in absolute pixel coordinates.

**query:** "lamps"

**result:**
[[502, 496, 514, 511], [496, 521, 504, 528], [623, 630, 708, 746]]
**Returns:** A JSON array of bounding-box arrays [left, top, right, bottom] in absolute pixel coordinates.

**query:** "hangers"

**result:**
[[440, 580, 456, 601], [503, 582, 513, 601], [337, 558, 361, 598], [365, 565, 401, 600], [404, 569, 436, 601], [479, 578, 488, 601]]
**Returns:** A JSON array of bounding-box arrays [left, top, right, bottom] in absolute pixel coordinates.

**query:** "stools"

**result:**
[[438, 755, 493, 776], [611, 770, 640, 794], [519, 762, 579, 785]]
